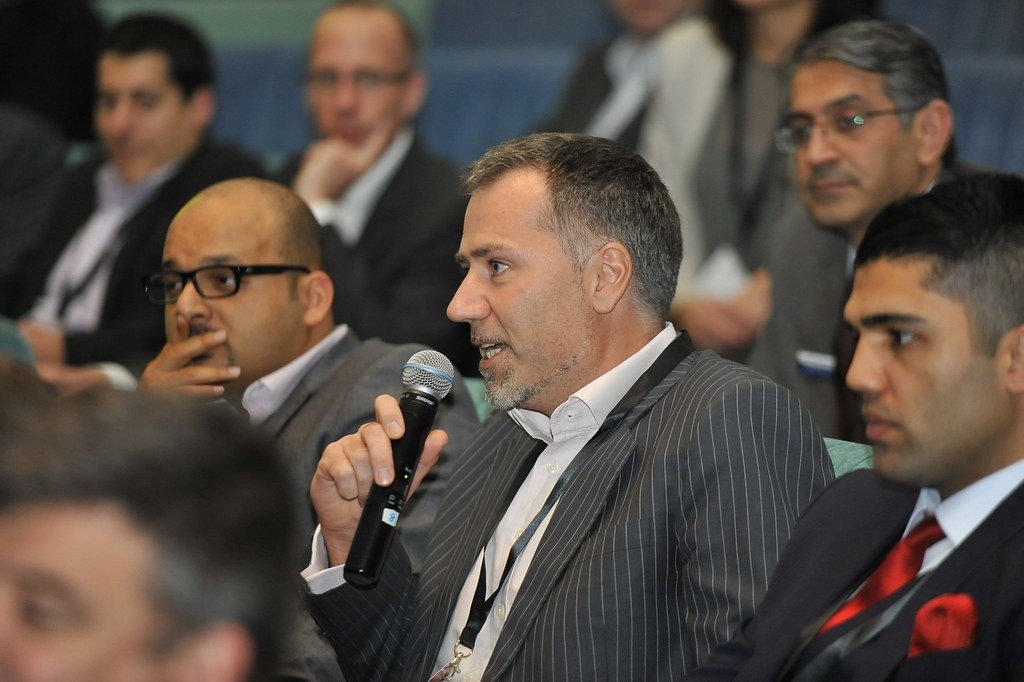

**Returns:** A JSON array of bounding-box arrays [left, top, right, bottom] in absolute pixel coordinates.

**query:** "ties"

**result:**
[[819, 514, 945, 634]]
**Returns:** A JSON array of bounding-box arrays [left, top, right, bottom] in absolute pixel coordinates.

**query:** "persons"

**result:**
[[545, 0, 888, 350], [686, 171, 1024, 682], [134, 178, 480, 682], [297, 132, 837, 682], [0, 387, 296, 682], [745, 16, 961, 452], [0, 13, 267, 397], [265, 0, 481, 379]]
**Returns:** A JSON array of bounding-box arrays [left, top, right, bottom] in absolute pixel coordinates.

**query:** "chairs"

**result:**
[[213, 0, 1024, 186]]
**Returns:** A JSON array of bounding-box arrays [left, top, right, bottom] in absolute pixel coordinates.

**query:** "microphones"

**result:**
[[344, 351, 454, 591]]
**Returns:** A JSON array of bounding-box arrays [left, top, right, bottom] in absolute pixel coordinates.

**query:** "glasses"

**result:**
[[143, 263, 309, 305], [300, 64, 412, 91], [773, 103, 939, 154]]
